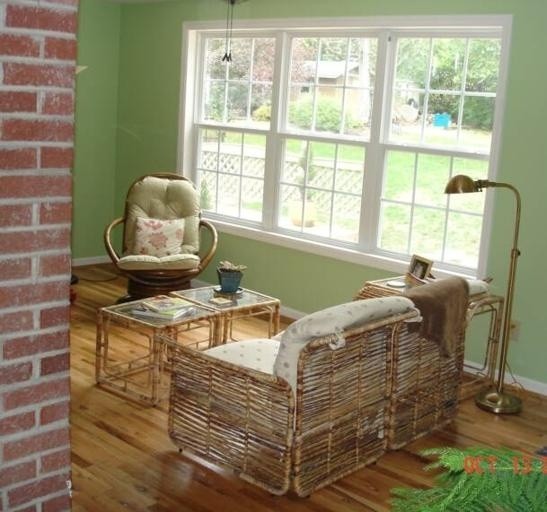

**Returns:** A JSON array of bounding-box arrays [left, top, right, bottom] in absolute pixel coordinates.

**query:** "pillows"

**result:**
[[117, 255, 201, 271], [132, 217, 185, 257], [123, 175, 201, 253]]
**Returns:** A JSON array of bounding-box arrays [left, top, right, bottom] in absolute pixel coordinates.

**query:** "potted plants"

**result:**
[[216, 257, 247, 292]]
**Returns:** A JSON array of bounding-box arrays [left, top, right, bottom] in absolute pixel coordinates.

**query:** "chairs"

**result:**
[[163, 305, 424, 497], [272, 276, 472, 451], [104, 173, 218, 302]]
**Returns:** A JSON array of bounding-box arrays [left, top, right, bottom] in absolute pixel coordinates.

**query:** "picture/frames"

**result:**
[[408, 253, 433, 280]]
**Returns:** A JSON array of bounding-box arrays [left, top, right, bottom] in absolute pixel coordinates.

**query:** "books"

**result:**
[[131, 297, 195, 320]]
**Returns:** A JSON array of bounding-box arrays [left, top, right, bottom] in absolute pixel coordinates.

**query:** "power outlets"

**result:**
[[508, 320, 520, 337]]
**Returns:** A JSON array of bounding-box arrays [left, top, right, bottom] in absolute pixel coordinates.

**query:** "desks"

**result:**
[[352, 274, 506, 400]]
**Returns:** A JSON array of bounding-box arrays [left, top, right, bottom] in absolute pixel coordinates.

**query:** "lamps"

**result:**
[[445, 174, 523, 414]]
